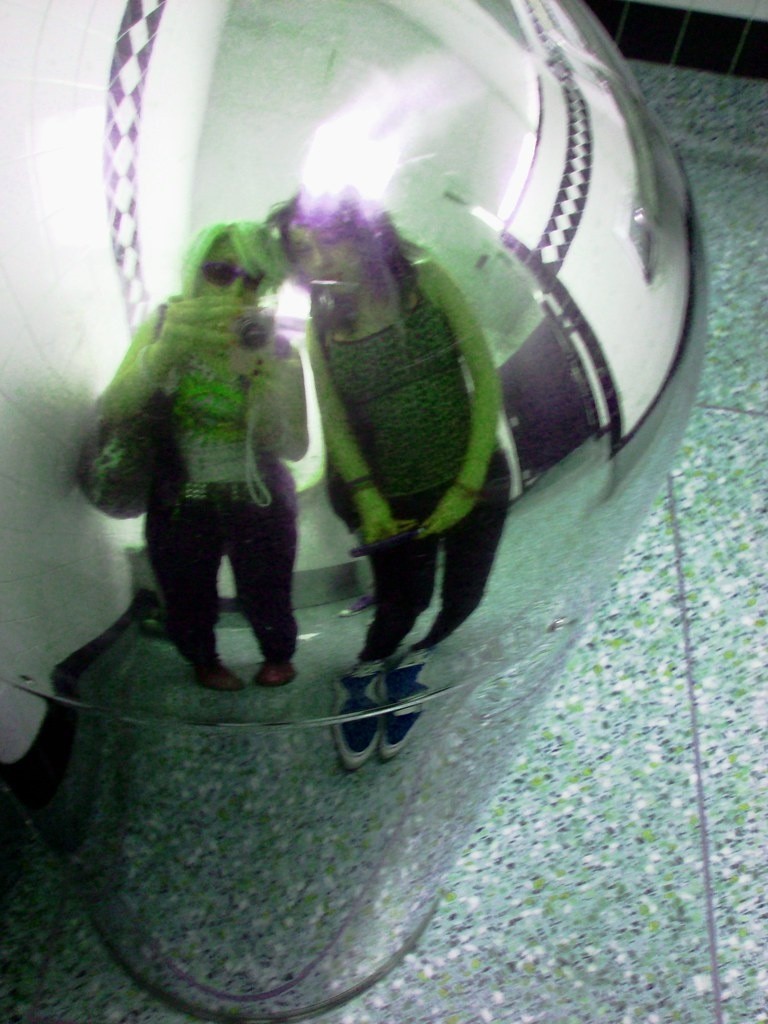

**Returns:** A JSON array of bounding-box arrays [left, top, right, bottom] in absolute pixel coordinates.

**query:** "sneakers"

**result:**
[[339, 593, 374, 618], [331, 663, 380, 769], [377, 647, 429, 762]]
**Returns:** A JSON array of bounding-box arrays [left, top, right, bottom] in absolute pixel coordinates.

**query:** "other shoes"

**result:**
[[252, 660, 295, 684], [192, 661, 247, 691]]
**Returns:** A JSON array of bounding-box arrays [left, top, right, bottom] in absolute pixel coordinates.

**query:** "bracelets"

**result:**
[[137, 346, 168, 388], [349, 475, 374, 494], [453, 480, 480, 499]]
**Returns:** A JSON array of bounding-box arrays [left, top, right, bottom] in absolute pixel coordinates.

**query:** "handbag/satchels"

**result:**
[[317, 325, 375, 533], [74, 304, 177, 520]]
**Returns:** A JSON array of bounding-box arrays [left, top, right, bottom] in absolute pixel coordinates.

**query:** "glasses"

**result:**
[[202, 263, 266, 292]]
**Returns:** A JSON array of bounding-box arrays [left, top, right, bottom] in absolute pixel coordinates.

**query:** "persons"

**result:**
[[100, 221, 307, 690], [278, 185, 524, 769]]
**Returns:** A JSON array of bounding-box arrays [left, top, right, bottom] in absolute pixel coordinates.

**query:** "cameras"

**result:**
[[225, 307, 275, 351]]
